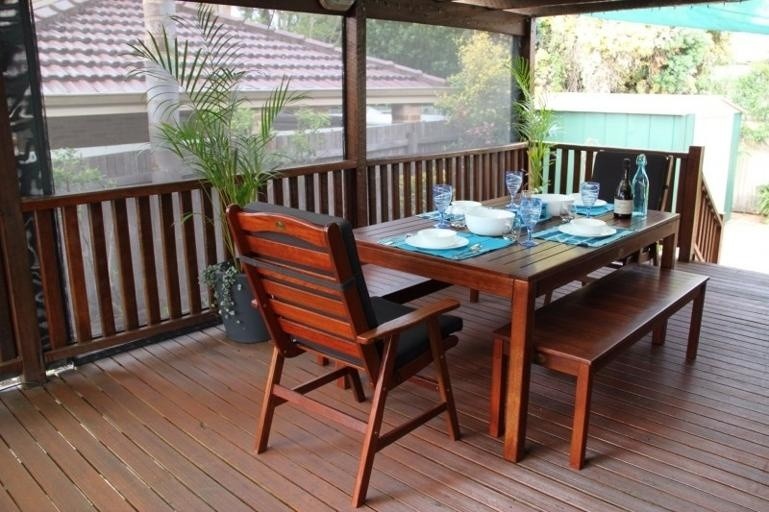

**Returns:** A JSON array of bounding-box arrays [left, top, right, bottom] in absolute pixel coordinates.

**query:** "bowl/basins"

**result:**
[[418, 229, 457, 247], [451, 200, 481, 213], [465, 206, 517, 236], [570, 217, 608, 231], [531, 192, 575, 215]]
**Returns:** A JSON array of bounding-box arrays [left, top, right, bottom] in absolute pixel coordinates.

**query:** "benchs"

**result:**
[[484, 257, 712, 471]]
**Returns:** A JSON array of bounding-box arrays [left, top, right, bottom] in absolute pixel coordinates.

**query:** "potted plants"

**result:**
[[121, 3, 315, 347]]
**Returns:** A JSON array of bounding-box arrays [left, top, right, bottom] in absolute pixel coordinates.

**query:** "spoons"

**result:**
[[453, 242, 481, 260]]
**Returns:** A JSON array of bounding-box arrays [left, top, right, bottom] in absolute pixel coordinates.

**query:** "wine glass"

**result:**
[[516, 196, 542, 247], [502, 168, 523, 209], [579, 182, 601, 216], [431, 183, 454, 229]]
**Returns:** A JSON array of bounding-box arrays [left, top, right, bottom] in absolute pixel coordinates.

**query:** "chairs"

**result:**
[[221, 198, 467, 509], [541, 147, 677, 308]]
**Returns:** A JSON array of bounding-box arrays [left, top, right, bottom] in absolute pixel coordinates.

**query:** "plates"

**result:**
[[559, 222, 617, 237], [568, 194, 606, 207], [405, 235, 470, 250]]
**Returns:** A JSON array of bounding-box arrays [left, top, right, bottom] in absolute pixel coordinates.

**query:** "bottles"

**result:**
[[632, 154, 650, 217], [612, 159, 634, 219]]
